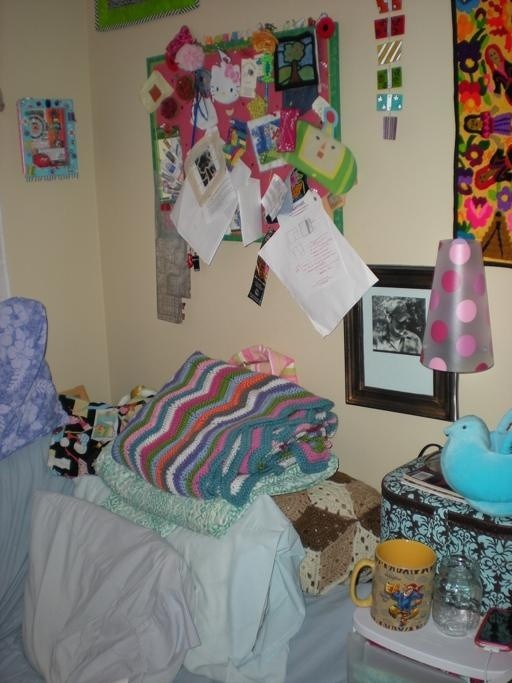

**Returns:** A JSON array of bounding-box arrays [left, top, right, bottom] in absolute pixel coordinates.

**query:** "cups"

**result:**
[[349, 539, 437, 631]]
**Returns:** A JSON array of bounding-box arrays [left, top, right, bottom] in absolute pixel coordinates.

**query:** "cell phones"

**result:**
[[474, 607, 512, 652]]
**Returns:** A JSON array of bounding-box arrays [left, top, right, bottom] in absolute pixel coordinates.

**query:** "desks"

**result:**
[[347, 594, 511, 683]]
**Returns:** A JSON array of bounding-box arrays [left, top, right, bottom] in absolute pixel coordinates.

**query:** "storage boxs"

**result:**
[[380, 452, 511, 616]]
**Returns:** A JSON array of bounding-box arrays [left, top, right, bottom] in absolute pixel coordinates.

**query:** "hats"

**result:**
[[385, 298, 410, 322]]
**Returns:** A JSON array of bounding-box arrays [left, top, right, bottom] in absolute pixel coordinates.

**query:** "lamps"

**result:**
[[417, 239, 494, 474]]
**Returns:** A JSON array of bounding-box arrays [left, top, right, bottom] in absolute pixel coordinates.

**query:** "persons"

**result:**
[[381, 299, 423, 354], [371, 307, 401, 352]]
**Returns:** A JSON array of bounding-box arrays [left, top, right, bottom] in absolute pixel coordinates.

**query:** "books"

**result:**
[[400, 477, 466, 503], [402, 465, 465, 498]]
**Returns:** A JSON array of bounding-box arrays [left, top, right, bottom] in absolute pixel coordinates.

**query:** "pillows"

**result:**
[[1, 294, 65, 462], [23, 486, 203, 682]]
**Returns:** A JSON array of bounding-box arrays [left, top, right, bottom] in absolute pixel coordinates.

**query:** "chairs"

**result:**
[[0, 434, 306, 683]]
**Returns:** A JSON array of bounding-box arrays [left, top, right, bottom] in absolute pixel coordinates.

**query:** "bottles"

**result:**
[[432, 554, 482, 636]]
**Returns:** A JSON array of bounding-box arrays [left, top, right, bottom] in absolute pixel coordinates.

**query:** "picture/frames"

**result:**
[[450, 0, 512, 268], [146, 20, 344, 242], [16, 96, 79, 182], [94, 0, 200, 32], [342, 265, 453, 422]]
